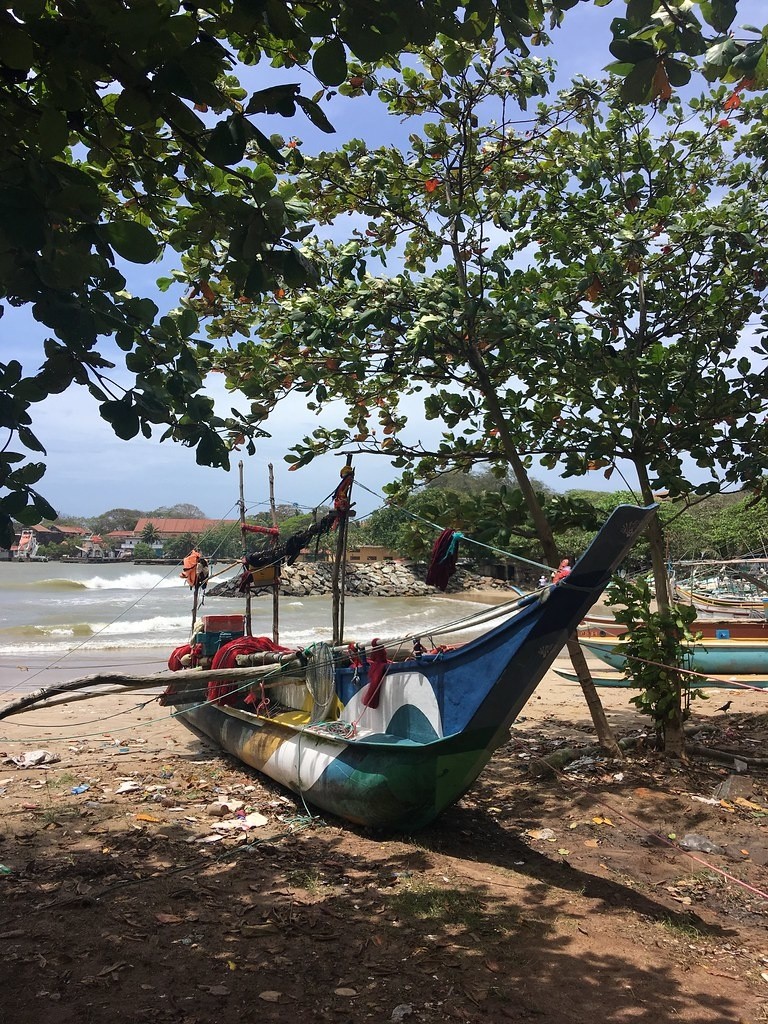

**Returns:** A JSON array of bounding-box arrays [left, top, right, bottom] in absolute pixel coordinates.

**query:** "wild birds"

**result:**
[[713, 701, 735, 713]]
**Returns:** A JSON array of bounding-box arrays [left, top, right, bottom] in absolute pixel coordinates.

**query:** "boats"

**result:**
[[168, 455, 660, 826], [551, 550, 768, 687]]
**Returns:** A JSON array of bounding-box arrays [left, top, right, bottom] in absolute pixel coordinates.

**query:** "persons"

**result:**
[[539, 576, 547, 587]]
